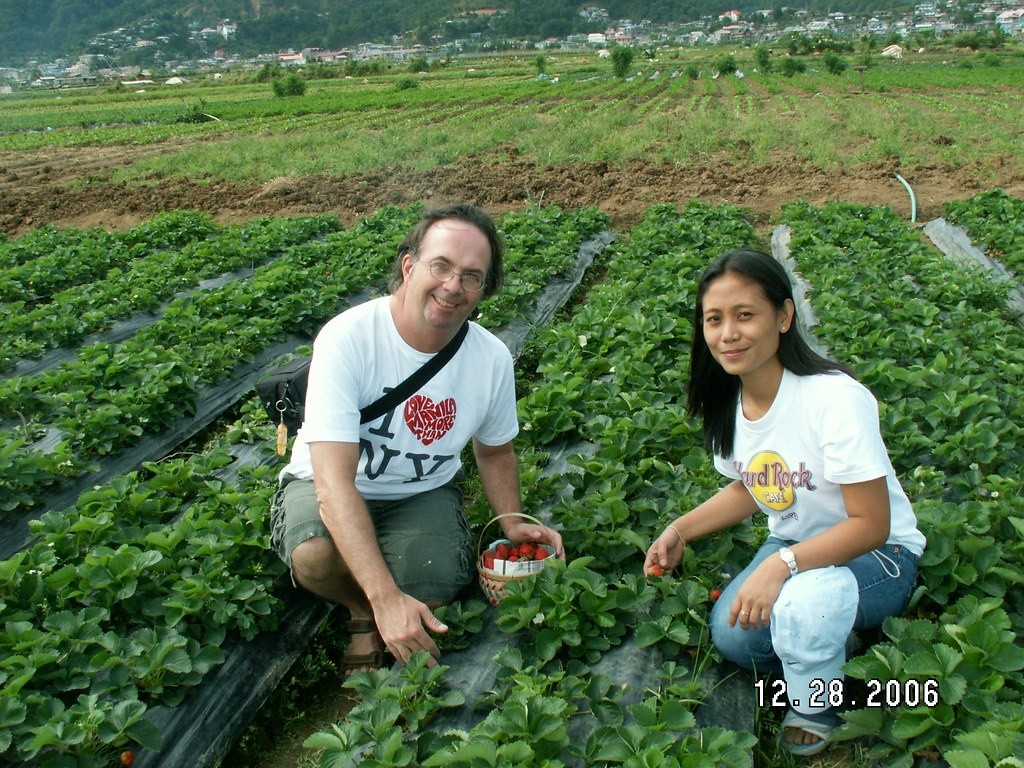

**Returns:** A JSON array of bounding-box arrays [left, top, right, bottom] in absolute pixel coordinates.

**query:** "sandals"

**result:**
[[338, 618, 384, 692]]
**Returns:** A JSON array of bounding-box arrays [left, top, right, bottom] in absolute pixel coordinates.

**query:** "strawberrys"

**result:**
[[647, 566, 662, 577], [481, 540, 549, 569], [711, 590, 721, 600]]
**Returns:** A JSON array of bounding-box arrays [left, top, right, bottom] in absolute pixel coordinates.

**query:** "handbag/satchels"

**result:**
[[255, 356, 312, 438]]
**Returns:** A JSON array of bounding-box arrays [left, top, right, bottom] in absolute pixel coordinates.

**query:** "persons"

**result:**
[[270, 204, 565, 684], [643, 248, 927, 755]]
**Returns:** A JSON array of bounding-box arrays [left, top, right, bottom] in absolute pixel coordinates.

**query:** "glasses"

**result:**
[[414, 254, 489, 292]]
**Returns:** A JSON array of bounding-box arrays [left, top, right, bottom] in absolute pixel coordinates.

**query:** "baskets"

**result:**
[[475, 511, 567, 607]]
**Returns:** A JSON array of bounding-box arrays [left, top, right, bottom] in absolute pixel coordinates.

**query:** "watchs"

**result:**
[[779, 547, 798, 575]]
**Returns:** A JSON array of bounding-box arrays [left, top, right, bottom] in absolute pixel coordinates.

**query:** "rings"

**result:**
[[741, 610, 749, 615]]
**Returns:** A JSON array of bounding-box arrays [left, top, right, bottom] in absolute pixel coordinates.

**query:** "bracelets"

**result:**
[[667, 526, 685, 546]]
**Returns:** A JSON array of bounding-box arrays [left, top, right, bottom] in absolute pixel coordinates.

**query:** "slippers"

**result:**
[[777, 706, 839, 757]]
